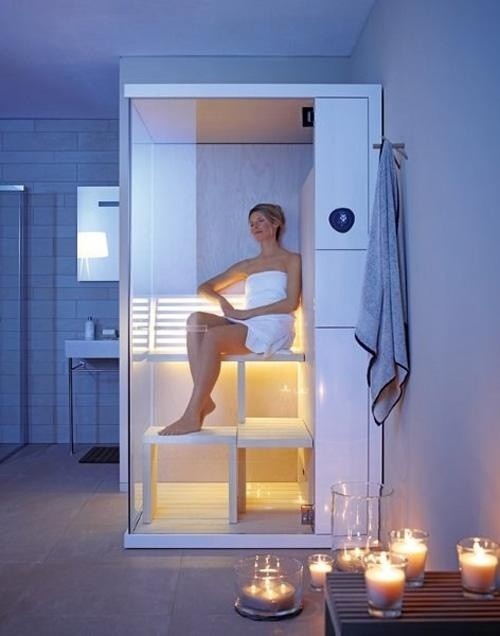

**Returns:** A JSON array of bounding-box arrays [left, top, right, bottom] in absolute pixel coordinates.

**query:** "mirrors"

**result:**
[[77, 186, 119, 281]]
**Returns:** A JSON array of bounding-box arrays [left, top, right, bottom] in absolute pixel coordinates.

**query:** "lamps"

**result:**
[[77, 233, 109, 278]]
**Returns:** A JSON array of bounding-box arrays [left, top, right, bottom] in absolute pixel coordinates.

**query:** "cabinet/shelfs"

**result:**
[[132, 352, 311, 529]]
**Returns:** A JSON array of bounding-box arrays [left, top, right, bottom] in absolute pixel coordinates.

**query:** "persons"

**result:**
[[155, 202, 304, 438]]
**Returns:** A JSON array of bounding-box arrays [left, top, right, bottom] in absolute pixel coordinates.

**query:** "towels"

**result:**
[[224, 272, 295, 351], [353, 140, 410, 424]]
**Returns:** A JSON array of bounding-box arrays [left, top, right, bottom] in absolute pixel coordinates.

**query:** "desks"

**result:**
[[322, 570, 500, 636]]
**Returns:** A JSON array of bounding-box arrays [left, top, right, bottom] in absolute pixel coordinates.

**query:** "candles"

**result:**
[[245, 581, 293, 609], [365, 566, 405, 606], [311, 564, 332, 586], [392, 544, 427, 575], [338, 549, 364, 570], [458, 551, 497, 590]]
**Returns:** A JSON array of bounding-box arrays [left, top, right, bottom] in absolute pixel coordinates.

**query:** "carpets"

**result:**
[[79, 446, 118, 464]]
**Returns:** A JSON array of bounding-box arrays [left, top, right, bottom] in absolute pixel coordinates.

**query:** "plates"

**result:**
[[235, 597, 304, 619]]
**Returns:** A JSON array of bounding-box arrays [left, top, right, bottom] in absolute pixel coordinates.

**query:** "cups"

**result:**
[[364, 553, 407, 617], [388, 527, 430, 586], [309, 554, 334, 591], [330, 482, 394, 573], [456, 536, 500, 600]]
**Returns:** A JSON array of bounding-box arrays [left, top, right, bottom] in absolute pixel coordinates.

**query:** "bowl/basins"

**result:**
[[233, 554, 303, 609]]
